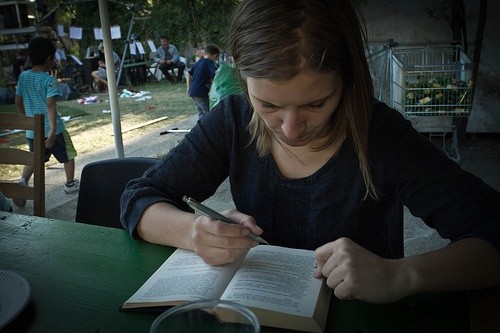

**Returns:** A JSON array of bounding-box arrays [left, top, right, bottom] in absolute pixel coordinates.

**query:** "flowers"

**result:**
[[404, 72, 473, 115]]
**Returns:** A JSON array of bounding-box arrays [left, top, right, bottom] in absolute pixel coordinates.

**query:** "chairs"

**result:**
[[0, 112, 45, 217], [146, 62, 179, 83], [75, 157, 161, 230]]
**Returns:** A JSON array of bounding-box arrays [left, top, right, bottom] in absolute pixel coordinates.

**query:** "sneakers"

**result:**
[[62, 179, 80, 193], [11, 179, 28, 208]]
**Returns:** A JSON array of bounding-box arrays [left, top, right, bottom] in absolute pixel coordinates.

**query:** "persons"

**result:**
[[153, 35, 186, 84], [184, 44, 219, 119], [120, 0, 500, 304], [15, 39, 121, 92], [11, 37, 81, 208]]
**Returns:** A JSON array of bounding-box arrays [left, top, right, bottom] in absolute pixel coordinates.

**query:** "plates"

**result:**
[[0, 270, 32, 329]]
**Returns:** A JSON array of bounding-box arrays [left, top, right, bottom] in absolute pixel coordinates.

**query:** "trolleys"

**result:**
[[351, 39, 399, 102], [388, 43, 474, 162]]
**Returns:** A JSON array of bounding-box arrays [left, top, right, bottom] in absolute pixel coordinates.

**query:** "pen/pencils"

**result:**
[[184, 196, 270, 245]]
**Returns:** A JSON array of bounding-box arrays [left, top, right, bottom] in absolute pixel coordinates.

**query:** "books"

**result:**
[[120, 243, 331, 332]]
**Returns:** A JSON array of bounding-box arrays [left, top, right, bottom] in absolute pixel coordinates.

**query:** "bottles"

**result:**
[[404, 76, 473, 117]]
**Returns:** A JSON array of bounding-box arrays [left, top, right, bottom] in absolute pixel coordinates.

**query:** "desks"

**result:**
[[0, 210, 499, 332], [122, 60, 154, 86]]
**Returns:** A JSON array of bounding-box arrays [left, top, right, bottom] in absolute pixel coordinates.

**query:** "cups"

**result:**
[[150, 299, 260, 333]]
